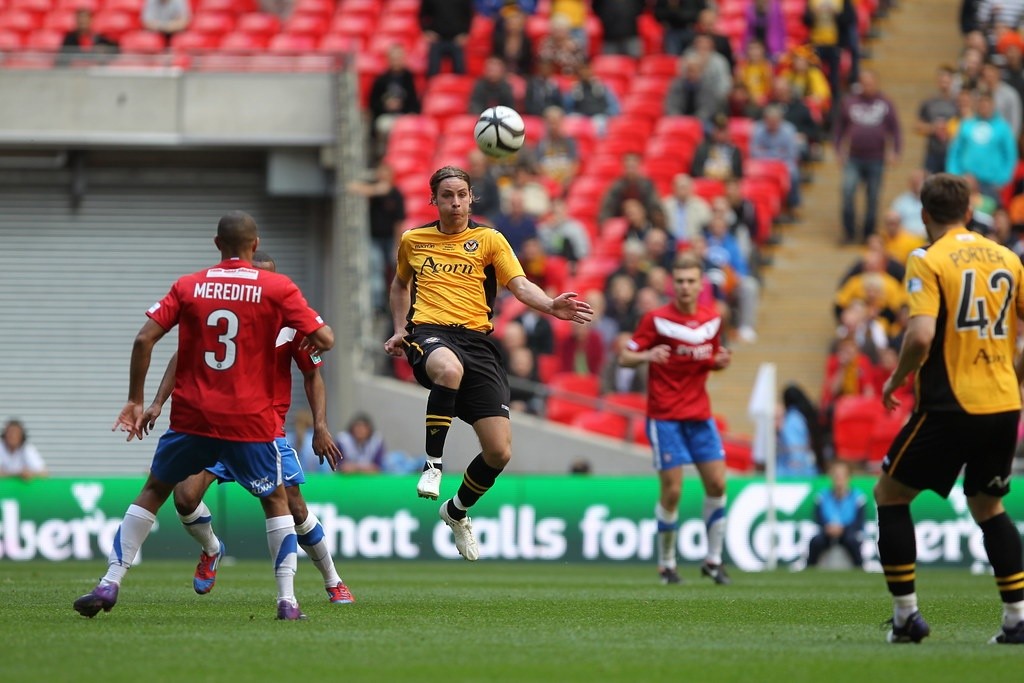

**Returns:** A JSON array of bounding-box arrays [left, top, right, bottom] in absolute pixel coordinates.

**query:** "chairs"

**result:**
[[0, 0, 1023, 463]]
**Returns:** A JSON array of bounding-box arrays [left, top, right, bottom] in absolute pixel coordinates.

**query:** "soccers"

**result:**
[[474, 106, 525, 158]]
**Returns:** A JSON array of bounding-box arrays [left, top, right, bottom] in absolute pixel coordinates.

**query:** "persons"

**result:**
[[55, 9, 120, 68], [805, 459, 867, 568], [140, 250, 355, 605], [383, 165, 594, 562], [292, 0, 1024, 481], [873, 173, 1023, 644], [617, 260, 736, 590], [0, 419, 48, 478], [74, 211, 335, 622], [140, 0, 193, 40]]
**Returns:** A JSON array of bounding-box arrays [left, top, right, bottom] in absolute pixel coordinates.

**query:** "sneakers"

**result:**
[[416, 459, 443, 501], [987, 620, 1024, 644], [880, 609, 930, 643], [439, 499, 480, 561], [702, 561, 731, 584], [325, 581, 355, 606], [657, 564, 682, 584], [193, 536, 226, 595], [277, 593, 306, 620], [73, 582, 119, 618]]
